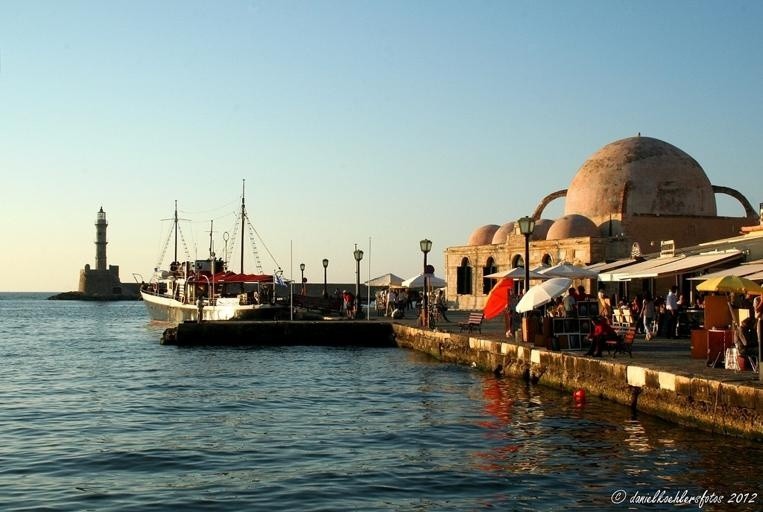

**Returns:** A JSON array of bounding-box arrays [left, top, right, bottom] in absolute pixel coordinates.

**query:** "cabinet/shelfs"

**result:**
[[706, 328, 732, 368]]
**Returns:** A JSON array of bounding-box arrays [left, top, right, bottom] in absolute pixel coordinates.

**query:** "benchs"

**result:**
[[457, 312, 483, 334], [604, 326, 636, 358]]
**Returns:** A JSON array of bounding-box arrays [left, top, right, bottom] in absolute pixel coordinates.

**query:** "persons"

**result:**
[[547, 285, 704, 358], [141, 280, 160, 293], [374, 289, 450, 322], [333, 287, 355, 320], [733, 317, 759, 357], [752, 295, 763, 362]]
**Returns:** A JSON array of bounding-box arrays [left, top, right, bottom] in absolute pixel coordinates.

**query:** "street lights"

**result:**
[[352, 249, 364, 320], [518, 215, 537, 317], [418, 237, 432, 325], [321, 257, 329, 298], [298, 262, 306, 294]]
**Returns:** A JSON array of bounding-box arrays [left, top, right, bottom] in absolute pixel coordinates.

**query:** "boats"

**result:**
[[135, 176, 290, 323]]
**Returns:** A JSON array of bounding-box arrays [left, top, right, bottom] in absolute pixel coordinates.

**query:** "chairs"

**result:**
[[611, 309, 622, 326], [621, 310, 634, 326]]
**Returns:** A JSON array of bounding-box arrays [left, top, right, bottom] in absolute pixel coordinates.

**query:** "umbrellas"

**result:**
[[515, 277, 573, 313], [483, 261, 597, 280], [696, 274, 763, 296], [483, 277, 515, 320]]
